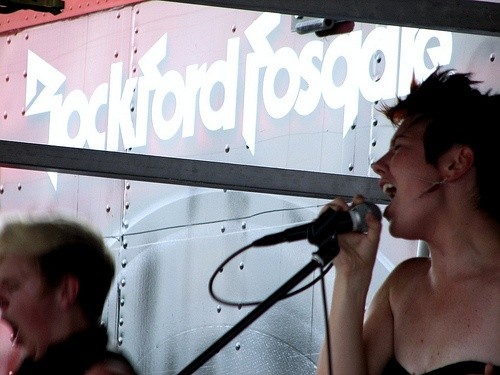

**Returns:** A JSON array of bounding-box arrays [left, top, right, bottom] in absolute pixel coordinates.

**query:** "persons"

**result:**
[[316, 64, 500, 375], [0, 220, 140, 375]]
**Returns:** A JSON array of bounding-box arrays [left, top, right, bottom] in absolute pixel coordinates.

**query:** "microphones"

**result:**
[[252, 201, 383, 246]]
